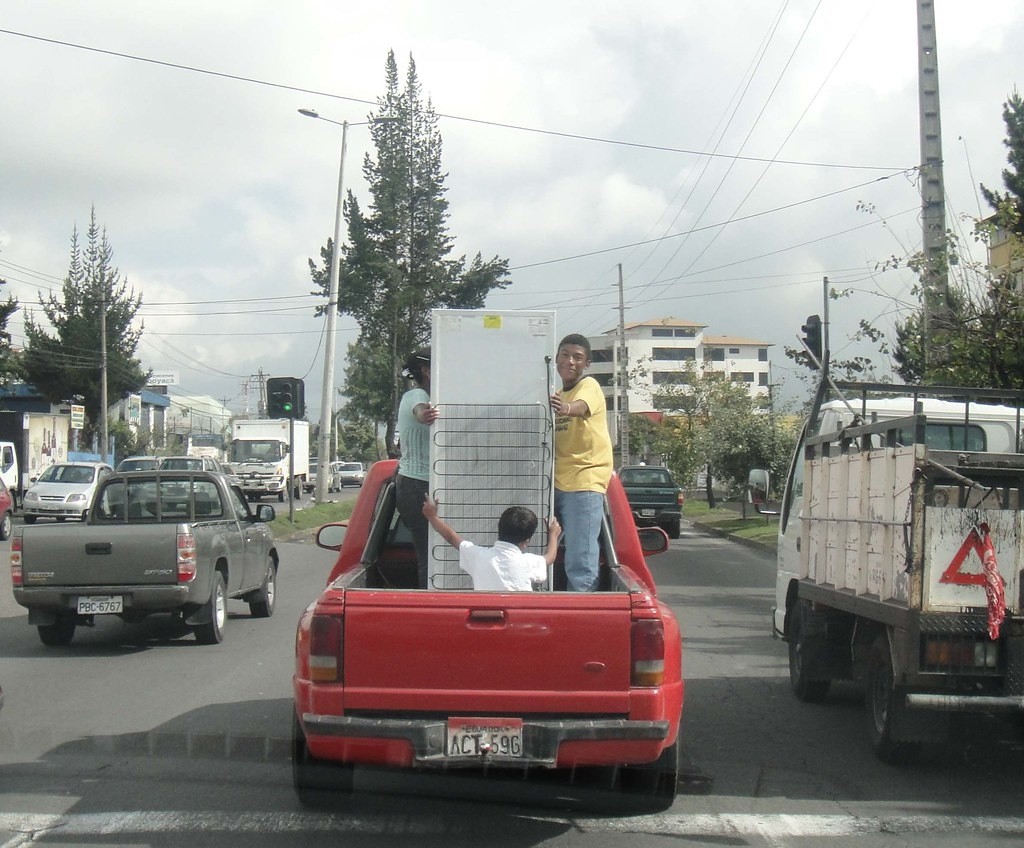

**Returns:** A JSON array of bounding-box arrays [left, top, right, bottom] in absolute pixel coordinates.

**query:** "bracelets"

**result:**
[[566, 402, 571, 415]]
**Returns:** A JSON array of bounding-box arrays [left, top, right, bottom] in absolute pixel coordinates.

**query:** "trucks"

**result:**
[[219, 418, 311, 502], [745, 345, 1024, 769], [0, 410, 73, 512]]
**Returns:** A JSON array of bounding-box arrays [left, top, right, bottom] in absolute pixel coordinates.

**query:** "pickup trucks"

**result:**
[[9, 469, 281, 644], [288, 458, 687, 812], [618, 464, 684, 538]]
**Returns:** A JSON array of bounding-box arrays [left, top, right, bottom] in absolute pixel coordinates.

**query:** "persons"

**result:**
[[550, 334, 614, 592], [422, 493, 562, 592], [395, 346, 440, 588]]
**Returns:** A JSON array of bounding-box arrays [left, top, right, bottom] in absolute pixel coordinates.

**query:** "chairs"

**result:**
[[114, 494, 143, 519], [187, 492, 212, 519]]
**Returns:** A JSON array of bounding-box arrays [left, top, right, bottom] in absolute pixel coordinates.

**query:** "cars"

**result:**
[[22, 461, 116, 523], [105, 455, 170, 512], [0, 479, 14, 540], [332, 460, 367, 487], [308, 464, 342, 495]]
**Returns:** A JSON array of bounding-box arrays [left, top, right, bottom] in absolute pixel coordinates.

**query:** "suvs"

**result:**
[[145, 454, 229, 516]]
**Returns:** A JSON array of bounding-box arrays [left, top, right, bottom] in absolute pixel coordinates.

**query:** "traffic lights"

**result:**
[[802, 316, 827, 371], [266, 377, 306, 418]]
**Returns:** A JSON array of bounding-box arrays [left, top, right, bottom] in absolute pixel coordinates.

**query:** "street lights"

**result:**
[[300, 106, 401, 513]]
